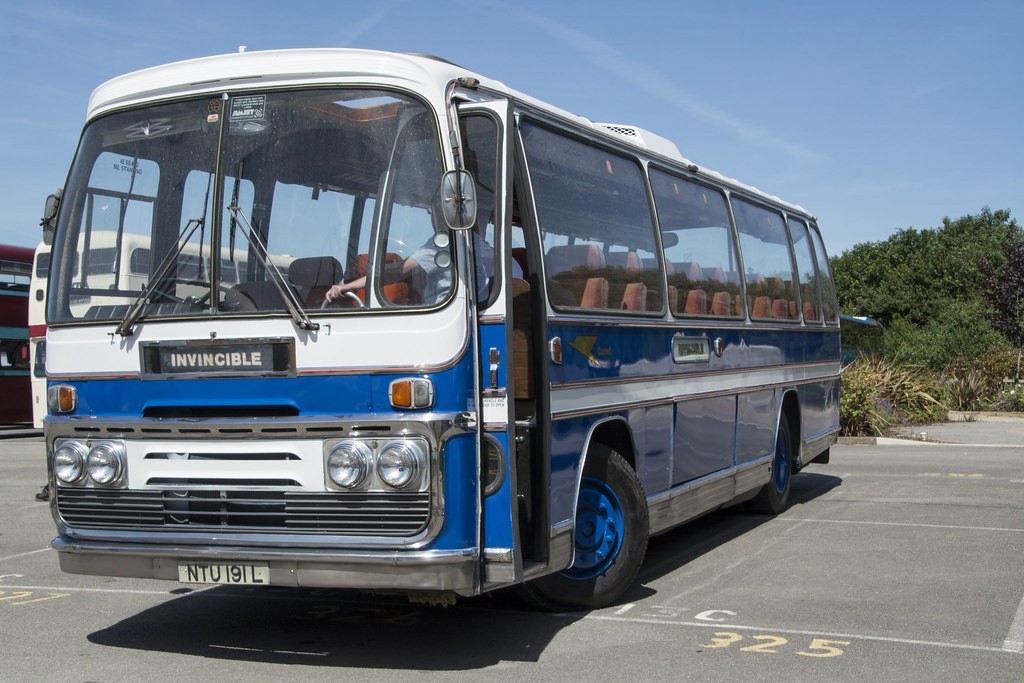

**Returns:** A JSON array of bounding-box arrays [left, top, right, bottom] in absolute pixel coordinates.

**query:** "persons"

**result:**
[[325, 198, 524, 312], [34, 484, 50, 502]]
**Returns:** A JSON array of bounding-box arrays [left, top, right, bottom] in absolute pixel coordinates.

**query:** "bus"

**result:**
[[43, 45, 843, 610], [25, 228, 297, 429], [0, 239, 36, 425]]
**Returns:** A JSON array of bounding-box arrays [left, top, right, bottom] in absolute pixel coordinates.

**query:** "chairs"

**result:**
[[784, 280, 798, 319], [225, 282, 303, 312], [343, 253, 408, 308], [766, 277, 788, 317], [547, 243, 608, 316], [641, 258, 679, 314], [725, 271, 750, 317], [604, 251, 647, 309], [672, 262, 707, 317], [702, 267, 732, 318], [800, 284, 815, 322], [289, 256, 343, 310], [511, 246, 529, 283], [746, 272, 773, 317]]
